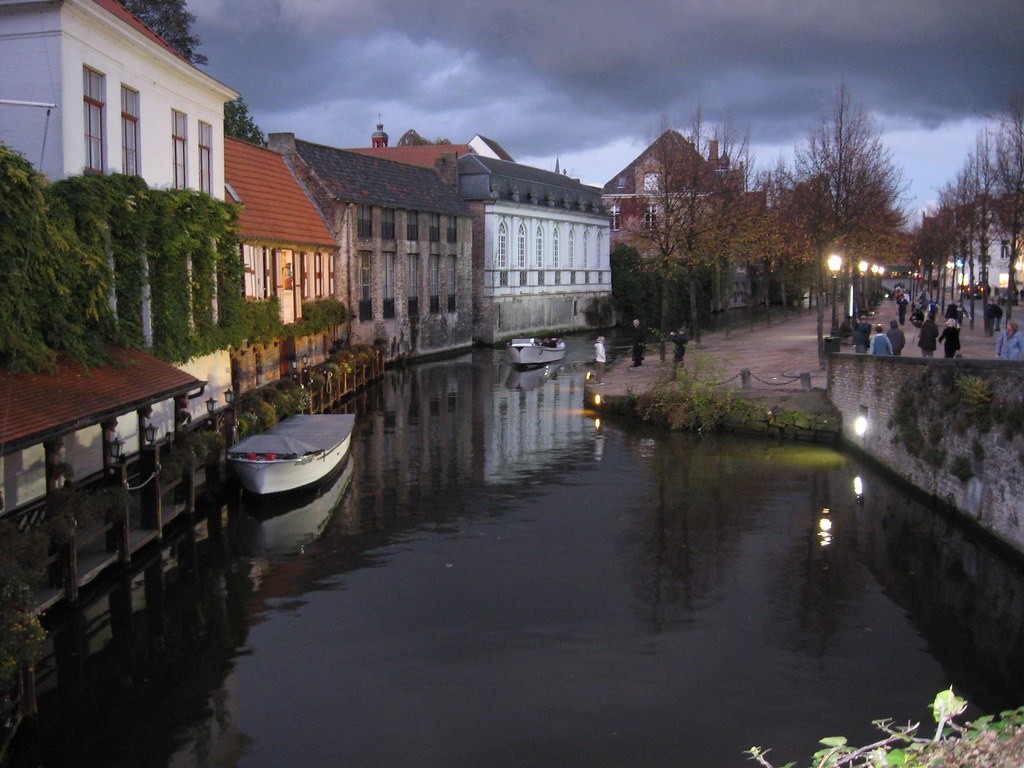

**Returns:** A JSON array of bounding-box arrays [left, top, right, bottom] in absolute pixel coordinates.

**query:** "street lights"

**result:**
[[827, 254, 842, 337], [870, 264, 878, 300], [858, 260, 868, 310]]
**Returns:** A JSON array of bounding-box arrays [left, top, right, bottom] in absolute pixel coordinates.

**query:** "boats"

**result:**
[[227, 449, 355, 559], [223, 411, 357, 501], [505, 337, 567, 367], [505, 359, 563, 392]]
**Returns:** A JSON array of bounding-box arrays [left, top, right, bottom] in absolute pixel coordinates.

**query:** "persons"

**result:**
[[987, 302, 1003, 331], [887, 320, 906, 355], [870, 325, 893, 356], [911, 310, 939, 358], [630, 318, 644, 366], [945, 299, 969, 326], [518, 333, 524, 339], [594, 336, 607, 385], [851, 314, 871, 353], [535, 336, 565, 348], [839, 315, 853, 336], [996, 319, 1024, 360], [670, 327, 688, 366], [891, 289, 939, 328], [938, 318, 960, 358]]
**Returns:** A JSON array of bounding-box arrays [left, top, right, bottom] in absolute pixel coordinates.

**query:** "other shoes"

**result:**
[[595, 380, 605, 386], [630, 363, 642, 367]]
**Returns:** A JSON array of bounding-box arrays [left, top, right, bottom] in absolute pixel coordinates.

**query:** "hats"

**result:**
[[677, 327, 686, 331], [860, 314, 867, 319]]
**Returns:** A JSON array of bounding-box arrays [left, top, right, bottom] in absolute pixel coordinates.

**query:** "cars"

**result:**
[[961, 283, 983, 300], [997, 291, 1018, 306]]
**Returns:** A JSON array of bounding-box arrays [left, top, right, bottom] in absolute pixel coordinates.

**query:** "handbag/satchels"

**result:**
[[956, 351, 962, 358]]
